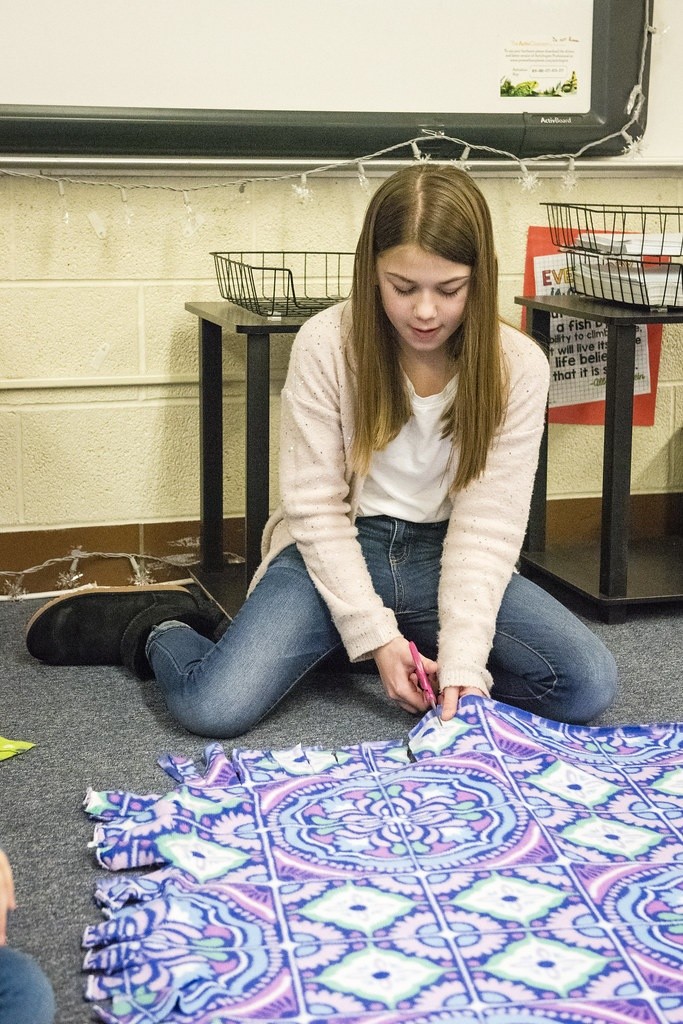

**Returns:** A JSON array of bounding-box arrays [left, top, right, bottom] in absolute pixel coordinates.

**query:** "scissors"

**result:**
[[407, 640, 444, 728]]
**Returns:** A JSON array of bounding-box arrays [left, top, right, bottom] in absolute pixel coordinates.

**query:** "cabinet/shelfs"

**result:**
[[183, 300, 314, 624], [512, 294, 682, 627]]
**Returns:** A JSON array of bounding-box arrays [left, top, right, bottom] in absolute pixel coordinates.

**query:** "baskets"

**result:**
[[209, 250, 356, 318], [540, 201, 683, 258], [564, 249, 683, 313]]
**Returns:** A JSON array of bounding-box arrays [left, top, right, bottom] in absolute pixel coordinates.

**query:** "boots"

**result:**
[[24, 583, 215, 681]]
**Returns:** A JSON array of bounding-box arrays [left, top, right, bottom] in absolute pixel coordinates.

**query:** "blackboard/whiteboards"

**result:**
[[0, 0, 655, 155]]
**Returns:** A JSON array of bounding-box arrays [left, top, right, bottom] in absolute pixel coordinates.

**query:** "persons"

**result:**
[[27, 162, 620, 739]]
